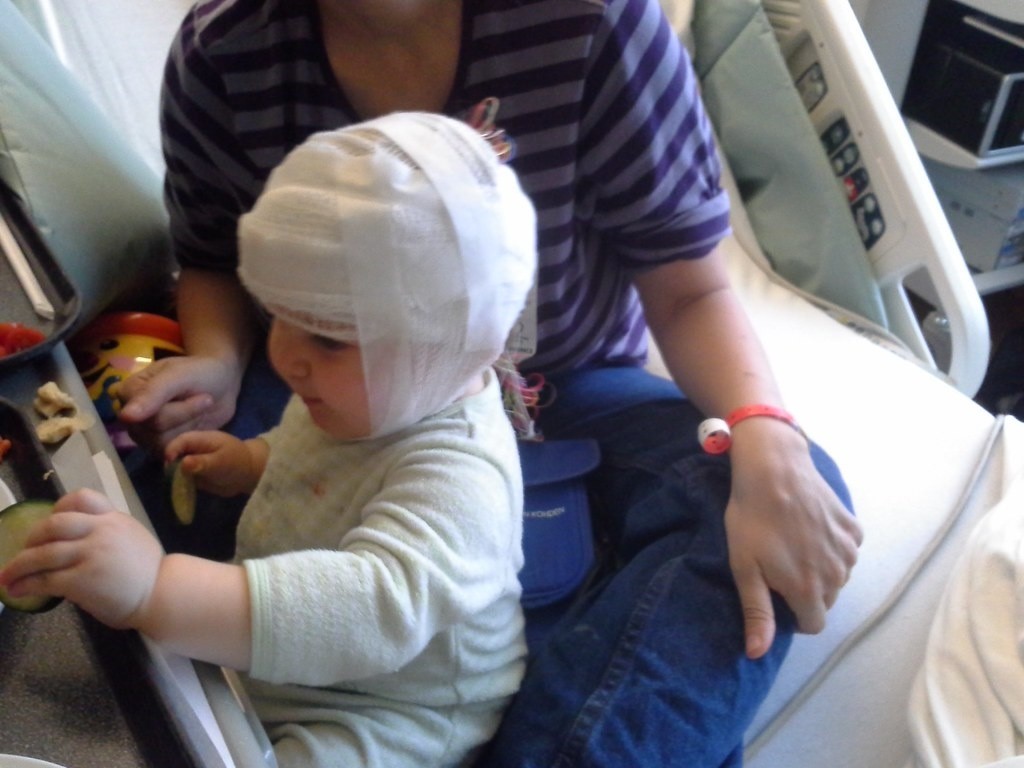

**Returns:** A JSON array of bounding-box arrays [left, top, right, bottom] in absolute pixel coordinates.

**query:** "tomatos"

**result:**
[[0, 320, 46, 357]]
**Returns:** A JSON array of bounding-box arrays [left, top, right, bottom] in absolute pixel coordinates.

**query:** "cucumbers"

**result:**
[[0, 498, 68, 614], [169, 459, 195, 525]]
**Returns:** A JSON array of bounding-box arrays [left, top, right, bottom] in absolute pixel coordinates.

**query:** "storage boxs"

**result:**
[[919, 155, 1023, 272]]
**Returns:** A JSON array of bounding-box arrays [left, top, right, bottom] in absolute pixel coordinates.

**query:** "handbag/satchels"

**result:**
[[518, 435, 603, 609]]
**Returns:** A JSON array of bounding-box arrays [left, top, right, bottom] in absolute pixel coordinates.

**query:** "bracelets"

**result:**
[[698, 405, 803, 454]]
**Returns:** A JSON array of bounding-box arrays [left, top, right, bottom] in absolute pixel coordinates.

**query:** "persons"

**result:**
[[0, 111, 536, 767], [119, 0, 863, 768]]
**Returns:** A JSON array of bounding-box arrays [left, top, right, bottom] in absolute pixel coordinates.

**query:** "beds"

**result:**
[[0, 0, 1024, 768]]
